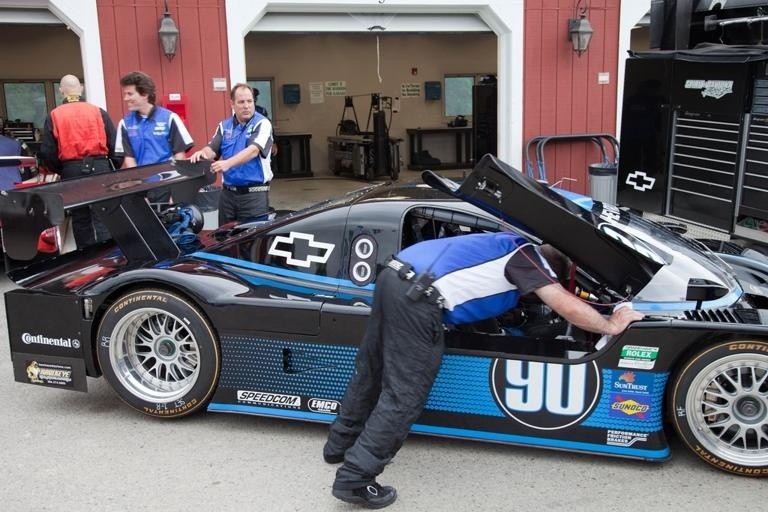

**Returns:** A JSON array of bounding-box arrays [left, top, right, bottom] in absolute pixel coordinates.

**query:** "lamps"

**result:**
[[159, 0, 182, 63], [566, 0, 593, 57]]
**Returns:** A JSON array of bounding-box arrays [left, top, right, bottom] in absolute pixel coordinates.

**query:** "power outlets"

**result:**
[[169, 94, 181, 101]]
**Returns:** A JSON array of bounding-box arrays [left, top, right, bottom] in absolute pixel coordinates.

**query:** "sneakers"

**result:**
[[324, 454, 346, 463], [332, 482, 397, 508]]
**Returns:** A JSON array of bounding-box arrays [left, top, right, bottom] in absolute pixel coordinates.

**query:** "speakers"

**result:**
[[283, 83, 301, 105], [425, 81, 442, 101]]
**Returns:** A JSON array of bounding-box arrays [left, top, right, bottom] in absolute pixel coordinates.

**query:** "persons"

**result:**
[[186, 83, 273, 227], [40, 74, 117, 250], [323, 232, 645, 510], [114, 72, 195, 210], [252, 88, 278, 156], [0, 117, 23, 191]]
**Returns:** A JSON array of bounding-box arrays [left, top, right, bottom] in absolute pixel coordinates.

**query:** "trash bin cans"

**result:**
[[588, 163, 618, 205]]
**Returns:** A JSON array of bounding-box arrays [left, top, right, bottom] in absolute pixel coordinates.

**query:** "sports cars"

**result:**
[[1, 160, 767, 479]]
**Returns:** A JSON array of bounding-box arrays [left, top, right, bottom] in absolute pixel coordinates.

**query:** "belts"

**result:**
[[224, 183, 271, 194], [387, 257, 444, 307]]
